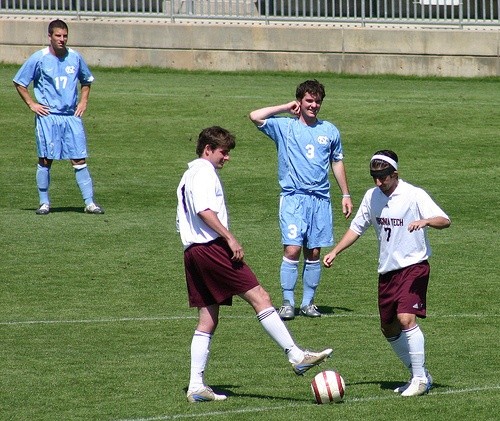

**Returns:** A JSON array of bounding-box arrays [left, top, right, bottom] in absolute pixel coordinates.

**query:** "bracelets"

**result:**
[[342, 195, 351, 198]]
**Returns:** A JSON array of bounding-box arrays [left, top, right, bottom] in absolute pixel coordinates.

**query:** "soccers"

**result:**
[[312, 371, 346, 404]]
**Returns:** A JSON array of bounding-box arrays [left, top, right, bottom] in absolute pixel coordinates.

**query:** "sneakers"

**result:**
[[394, 374, 433, 392], [290, 349, 334, 376], [84, 204, 104, 214], [401, 378, 430, 397], [298, 304, 321, 317], [187, 386, 227, 402], [278, 300, 294, 318], [36, 204, 49, 214]]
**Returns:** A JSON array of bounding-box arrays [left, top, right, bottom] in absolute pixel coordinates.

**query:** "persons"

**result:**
[[13, 20, 105, 215], [176, 125, 333, 403], [324, 150, 452, 395], [250, 79, 352, 319]]
